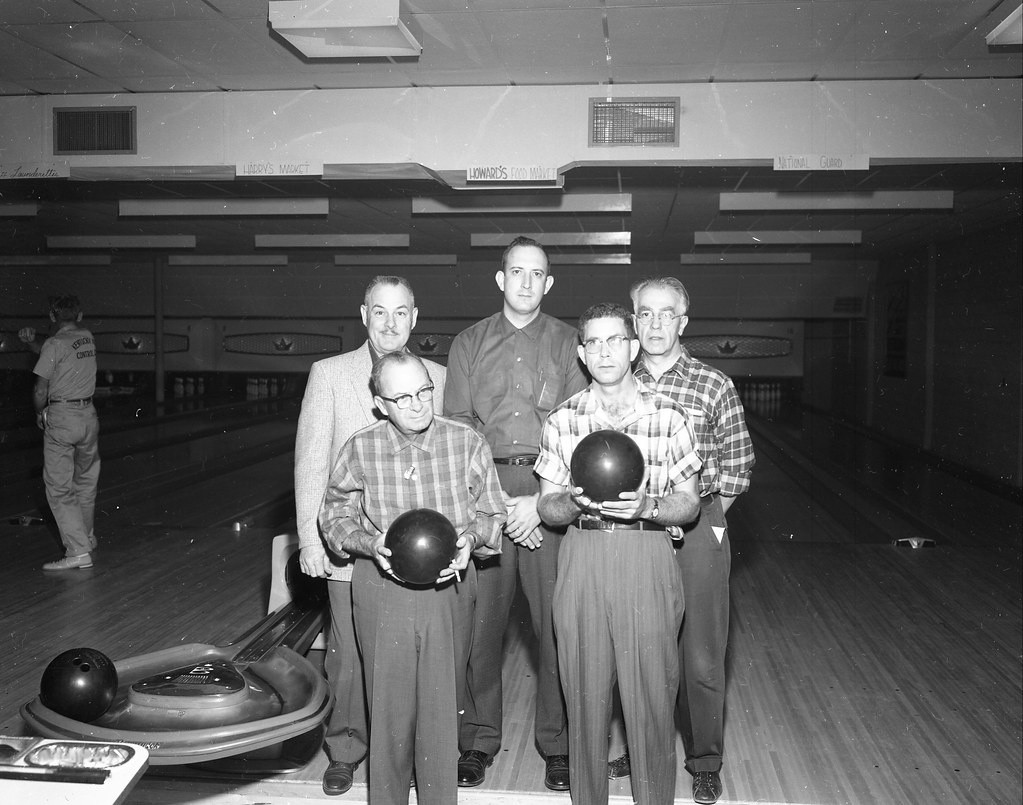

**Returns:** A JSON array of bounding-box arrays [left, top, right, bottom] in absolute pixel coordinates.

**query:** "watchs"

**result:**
[[651, 498, 659, 519]]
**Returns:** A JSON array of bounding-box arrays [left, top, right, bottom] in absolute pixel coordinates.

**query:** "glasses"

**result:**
[[635, 314, 682, 325], [584, 335, 631, 354], [375, 380, 434, 408]]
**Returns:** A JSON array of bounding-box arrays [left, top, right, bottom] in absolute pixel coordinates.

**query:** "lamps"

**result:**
[[986, 0, 1023, 46], [268, 0, 425, 58]]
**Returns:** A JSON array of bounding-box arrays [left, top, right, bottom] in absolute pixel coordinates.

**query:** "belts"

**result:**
[[572, 519, 667, 531], [49, 397, 91, 402], [492, 458, 537, 466], [699, 491, 721, 507]]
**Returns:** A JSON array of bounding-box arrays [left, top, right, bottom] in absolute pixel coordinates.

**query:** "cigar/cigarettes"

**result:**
[[452, 559, 461, 582]]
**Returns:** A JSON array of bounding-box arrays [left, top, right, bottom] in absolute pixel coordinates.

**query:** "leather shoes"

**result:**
[[43, 554, 92, 570], [323, 759, 358, 793], [458, 749, 493, 786], [544, 754, 570, 789], [409, 771, 417, 787], [692, 771, 722, 804], [608, 753, 630, 779]]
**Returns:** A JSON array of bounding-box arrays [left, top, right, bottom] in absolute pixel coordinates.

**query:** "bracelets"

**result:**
[[37, 412, 42, 415], [466, 531, 477, 543]]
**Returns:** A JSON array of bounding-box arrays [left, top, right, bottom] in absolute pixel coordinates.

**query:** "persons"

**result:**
[[318, 352, 509, 802], [295, 275, 447, 780], [27, 297, 102, 568], [443, 239, 592, 786], [609, 275, 757, 802], [533, 303, 701, 805]]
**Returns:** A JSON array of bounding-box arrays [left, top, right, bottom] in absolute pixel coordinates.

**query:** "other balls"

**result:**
[[39, 647, 120, 721], [384, 507, 460, 585], [569, 430, 646, 502]]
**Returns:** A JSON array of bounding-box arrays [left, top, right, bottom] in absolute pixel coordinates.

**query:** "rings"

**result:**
[[517, 528, 523, 534]]
